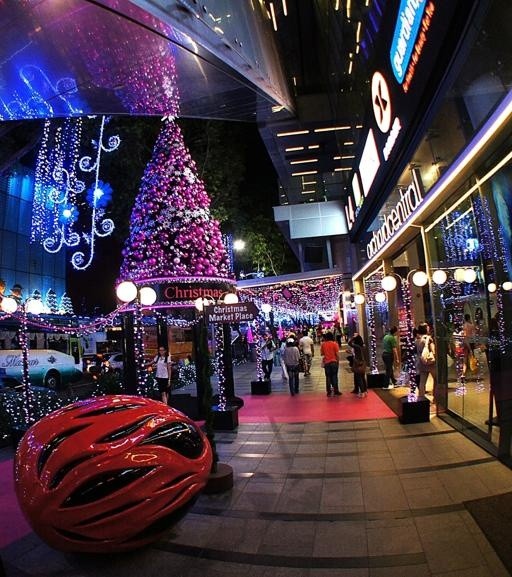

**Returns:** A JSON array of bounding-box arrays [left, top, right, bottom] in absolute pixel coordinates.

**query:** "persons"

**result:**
[[255, 307, 487, 403], [143, 343, 172, 408]]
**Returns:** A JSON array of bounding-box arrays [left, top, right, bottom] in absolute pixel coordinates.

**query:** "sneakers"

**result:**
[[358, 392, 367, 398], [351, 390, 358, 393], [334, 391, 342, 395], [327, 391, 332, 396]]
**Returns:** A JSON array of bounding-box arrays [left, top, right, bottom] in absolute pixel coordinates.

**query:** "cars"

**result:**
[[82, 350, 125, 378]]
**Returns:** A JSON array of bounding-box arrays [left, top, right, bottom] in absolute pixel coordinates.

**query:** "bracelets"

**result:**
[[168, 378, 172, 382]]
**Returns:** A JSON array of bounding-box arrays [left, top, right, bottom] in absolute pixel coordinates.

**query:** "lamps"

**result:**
[[195, 291, 239, 312], [354, 267, 428, 304]]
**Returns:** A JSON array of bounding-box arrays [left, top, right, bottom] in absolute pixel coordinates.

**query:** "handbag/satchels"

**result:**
[[421, 346, 435, 365], [352, 358, 366, 375]]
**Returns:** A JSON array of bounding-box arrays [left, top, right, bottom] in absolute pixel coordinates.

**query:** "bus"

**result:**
[[140, 325, 216, 363], [1, 313, 92, 390]]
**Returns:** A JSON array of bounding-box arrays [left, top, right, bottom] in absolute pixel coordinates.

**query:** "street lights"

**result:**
[[249, 299, 273, 395], [2, 292, 44, 431], [116, 278, 158, 395], [380, 264, 431, 425], [354, 289, 393, 389], [434, 266, 512, 397], [195, 288, 243, 430]]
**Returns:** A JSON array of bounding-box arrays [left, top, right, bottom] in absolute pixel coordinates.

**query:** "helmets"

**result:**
[[14, 395, 214, 553]]
